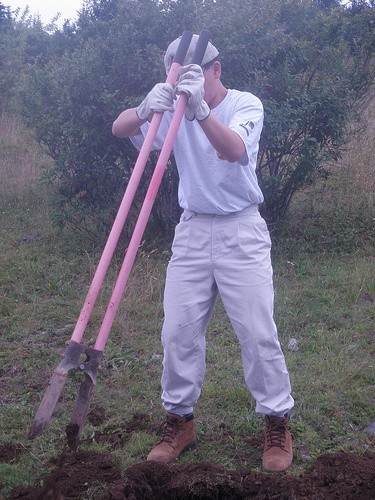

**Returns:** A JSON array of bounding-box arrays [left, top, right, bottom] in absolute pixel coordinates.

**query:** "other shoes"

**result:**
[[145, 410, 198, 468], [259, 411, 294, 474]]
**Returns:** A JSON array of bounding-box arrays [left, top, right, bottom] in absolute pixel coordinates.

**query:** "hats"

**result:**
[[161, 33, 220, 78]]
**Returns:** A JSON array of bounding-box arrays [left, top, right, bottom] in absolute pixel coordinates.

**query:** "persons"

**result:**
[[112, 35, 295, 472]]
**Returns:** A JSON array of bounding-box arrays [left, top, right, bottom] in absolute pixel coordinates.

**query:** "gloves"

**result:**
[[135, 81, 176, 122], [174, 63, 212, 122]]
